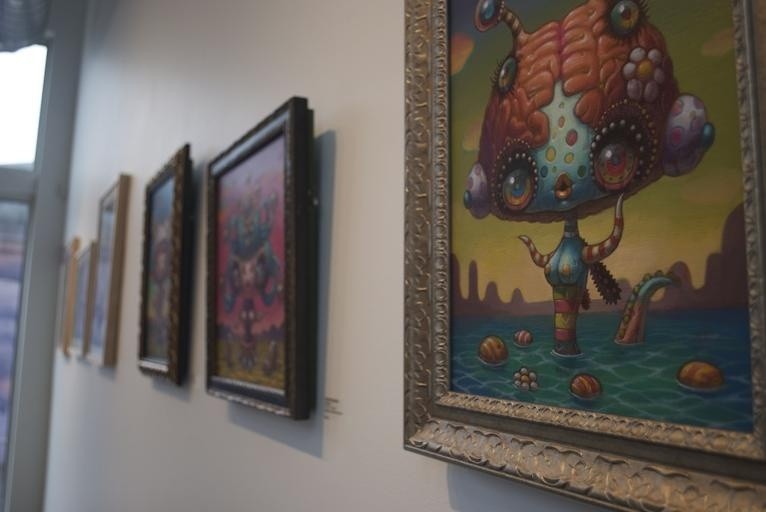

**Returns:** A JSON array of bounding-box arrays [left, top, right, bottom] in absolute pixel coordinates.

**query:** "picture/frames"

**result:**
[[136, 141, 188, 386], [402, 0, 766, 511], [200, 92, 320, 423], [53, 170, 130, 366]]
